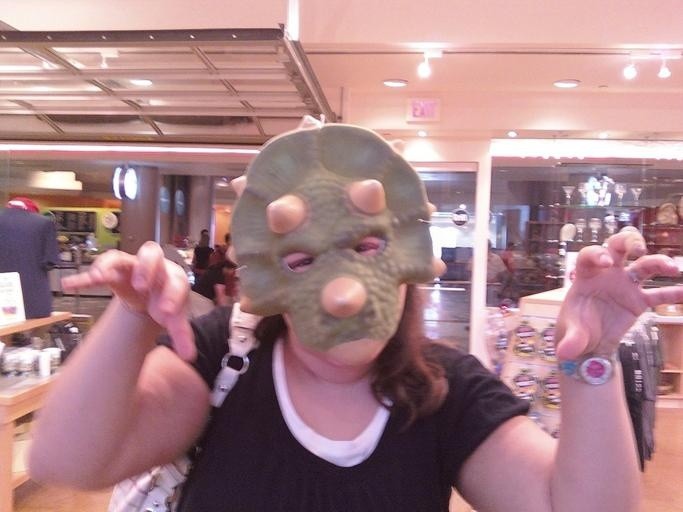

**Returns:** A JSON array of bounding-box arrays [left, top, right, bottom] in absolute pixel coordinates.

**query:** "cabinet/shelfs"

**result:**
[[0, 308, 77, 510], [522, 219, 640, 288]]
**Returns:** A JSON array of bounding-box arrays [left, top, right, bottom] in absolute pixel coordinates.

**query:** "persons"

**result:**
[[497, 243, 517, 300], [464, 239, 513, 330], [190, 228, 241, 308], [24, 115, 683, 511], [0, 197, 62, 318]]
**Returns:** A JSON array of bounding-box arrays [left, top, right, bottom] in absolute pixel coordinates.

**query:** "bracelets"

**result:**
[[551, 347, 622, 385]]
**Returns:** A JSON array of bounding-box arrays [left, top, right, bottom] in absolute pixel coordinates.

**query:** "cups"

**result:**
[[38, 347, 60, 377]]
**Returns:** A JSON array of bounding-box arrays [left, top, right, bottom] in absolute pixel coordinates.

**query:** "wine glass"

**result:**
[[562, 183, 642, 205]]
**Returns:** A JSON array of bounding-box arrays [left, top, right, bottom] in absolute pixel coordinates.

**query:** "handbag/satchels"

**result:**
[[108, 457, 192, 512]]
